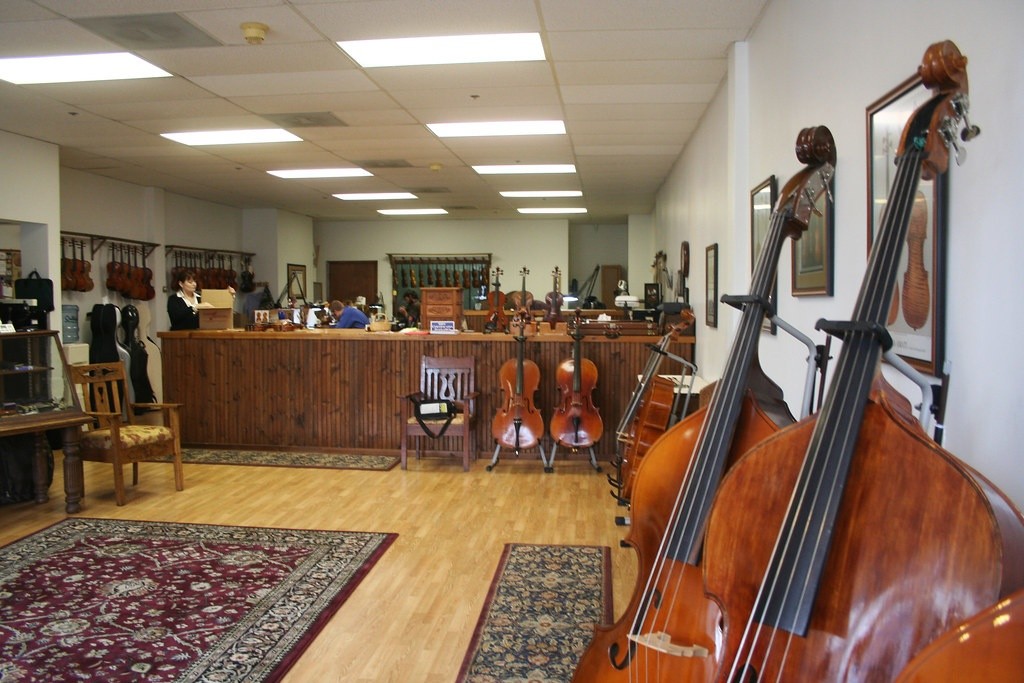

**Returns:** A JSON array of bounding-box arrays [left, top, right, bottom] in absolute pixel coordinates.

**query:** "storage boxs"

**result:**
[[253, 306, 329, 326], [511, 321, 568, 336], [197, 289, 234, 331]]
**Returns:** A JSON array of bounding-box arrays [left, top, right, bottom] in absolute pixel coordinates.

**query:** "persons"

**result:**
[[167, 268, 236, 331], [344, 298, 353, 306], [399, 290, 421, 328], [329, 300, 369, 329], [583, 296, 605, 309]]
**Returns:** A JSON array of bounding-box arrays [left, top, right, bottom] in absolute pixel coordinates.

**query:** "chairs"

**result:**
[[396, 353, 480, 472], [67, 361, 184, 507]]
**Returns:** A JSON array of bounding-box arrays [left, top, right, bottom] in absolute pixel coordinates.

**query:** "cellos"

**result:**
[[486, 267, 564, 328], [695, 38, 1024, 683], [491, 307, 545, 468], [611, 308, 697, 505], [548, 315, 604, 474], [572, 126, 838, 683]]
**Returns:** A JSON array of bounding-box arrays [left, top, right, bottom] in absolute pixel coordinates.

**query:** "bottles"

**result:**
[[61, 304, 80, 343]]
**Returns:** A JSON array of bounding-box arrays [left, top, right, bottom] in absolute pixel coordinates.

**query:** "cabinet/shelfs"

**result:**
[[0, 329, 95, 514], [421, 288, 464, 330]]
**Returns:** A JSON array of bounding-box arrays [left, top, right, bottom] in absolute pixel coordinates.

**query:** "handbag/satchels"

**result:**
[[15, 271, 55, 311]]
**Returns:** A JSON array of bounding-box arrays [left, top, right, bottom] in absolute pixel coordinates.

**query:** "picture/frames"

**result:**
[[791, 177, 834, 297], [866, 74, 949, 379], [706, 243, 717, 328], [287, 264, 307, 299], [749, 175, 777, 335]]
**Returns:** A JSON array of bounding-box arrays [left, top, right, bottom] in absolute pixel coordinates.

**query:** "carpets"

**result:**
[[142, 448, 399, 472], [455, 543, 614, 683], [0, 516, 399, 683]]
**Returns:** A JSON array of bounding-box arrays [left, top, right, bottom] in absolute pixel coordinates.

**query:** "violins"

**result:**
[[59, 236, 256, 302], [392, 257, 490, 292]]
